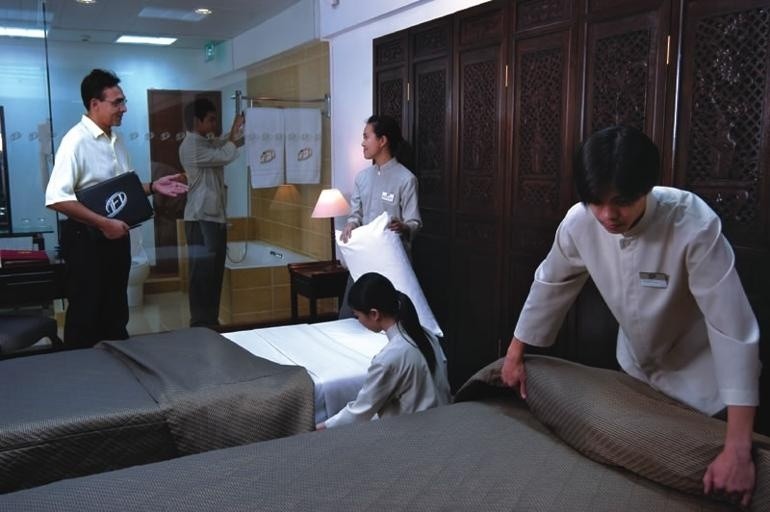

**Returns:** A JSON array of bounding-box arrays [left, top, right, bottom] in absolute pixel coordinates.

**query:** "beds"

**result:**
[[2, 314, 417, 493], [2, 351, 770, 512]]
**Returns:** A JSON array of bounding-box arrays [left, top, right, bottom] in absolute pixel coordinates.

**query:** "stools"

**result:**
[[1, 306, 64, 356]]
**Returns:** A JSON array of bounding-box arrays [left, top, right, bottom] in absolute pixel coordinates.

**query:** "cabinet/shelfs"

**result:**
[[369, 1, 769, 430]]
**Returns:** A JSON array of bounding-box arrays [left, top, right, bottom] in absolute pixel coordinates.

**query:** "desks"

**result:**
[[286, 259, 349, 319], [2, 226, 55, 253]]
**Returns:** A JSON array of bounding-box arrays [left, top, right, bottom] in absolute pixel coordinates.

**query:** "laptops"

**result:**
[[75, 170, 153, 227]]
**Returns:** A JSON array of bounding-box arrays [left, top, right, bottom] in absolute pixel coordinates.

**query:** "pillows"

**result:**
[[330, 210, 447, 342]]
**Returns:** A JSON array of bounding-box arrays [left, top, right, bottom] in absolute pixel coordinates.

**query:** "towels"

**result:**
[[243, 106, 286, 191], [282, 104, 323, 186]]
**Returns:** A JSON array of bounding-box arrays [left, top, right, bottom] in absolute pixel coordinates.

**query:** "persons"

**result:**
[[45, 69, 190, 351], [499, 126, 765, 508], [338, 114, 425, 318], [178, 99, 245, 328], [315, 272, 453, 431]]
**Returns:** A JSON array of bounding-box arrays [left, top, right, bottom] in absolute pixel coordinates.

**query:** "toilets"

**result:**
[[126, 224, 150, 306]]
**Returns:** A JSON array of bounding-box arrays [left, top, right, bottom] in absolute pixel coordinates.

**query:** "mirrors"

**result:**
[[0, 106, 15, 236]]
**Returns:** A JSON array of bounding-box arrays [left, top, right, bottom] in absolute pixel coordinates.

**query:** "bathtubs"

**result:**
[[217, 239, 323, 269]]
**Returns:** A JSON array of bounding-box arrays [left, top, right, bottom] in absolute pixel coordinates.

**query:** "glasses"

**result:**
[[100, 98, 128, 106]]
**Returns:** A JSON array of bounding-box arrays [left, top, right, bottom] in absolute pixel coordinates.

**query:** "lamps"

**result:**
[[310, 188, 352, 264]]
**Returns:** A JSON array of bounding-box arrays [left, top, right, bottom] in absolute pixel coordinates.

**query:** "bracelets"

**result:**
[[150, 181, 154, 194]]
